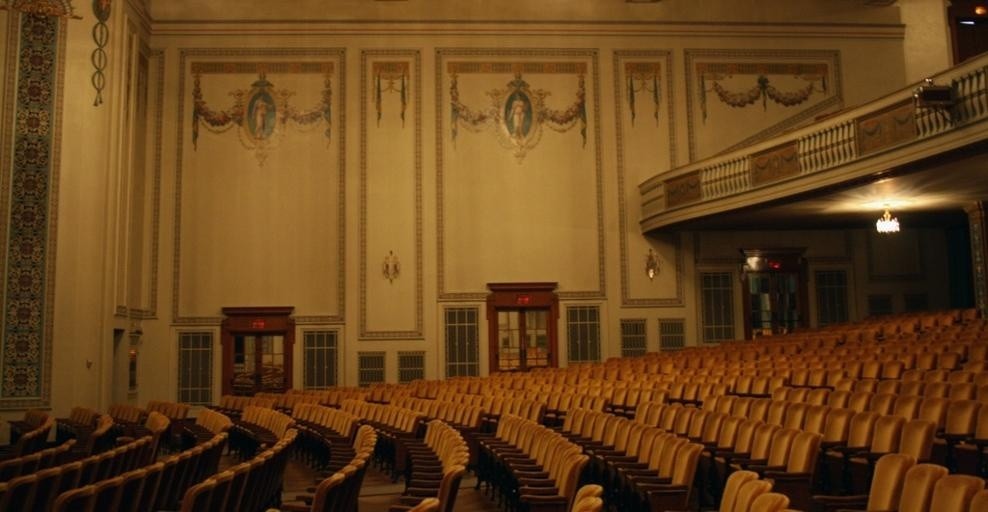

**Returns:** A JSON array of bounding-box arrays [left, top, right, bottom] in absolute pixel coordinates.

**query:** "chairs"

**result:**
[[1, 308, 988, 511]]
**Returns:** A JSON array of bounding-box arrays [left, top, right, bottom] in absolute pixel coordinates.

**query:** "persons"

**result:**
[[507, 92, 526, 137], [646, 249, 661, 277], [383, 250, 400, 275]]
